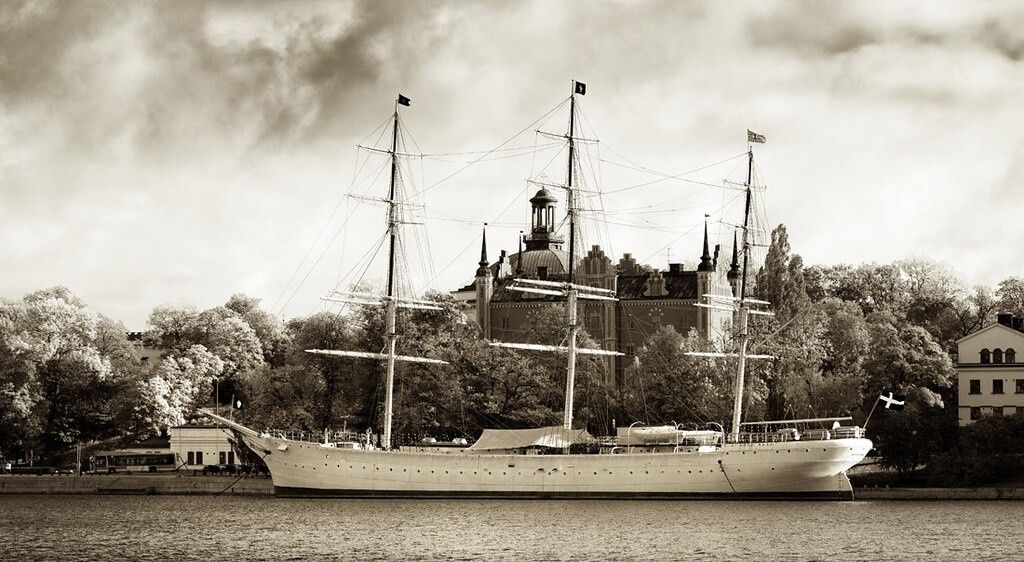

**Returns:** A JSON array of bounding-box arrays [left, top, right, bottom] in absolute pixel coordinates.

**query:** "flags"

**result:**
[[575, 81, 587, 95], [397, 93, 412, 107], [746, 129, 767, 145]]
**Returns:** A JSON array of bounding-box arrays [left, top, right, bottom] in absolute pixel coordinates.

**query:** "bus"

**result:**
[[93, 449, 177, 474]]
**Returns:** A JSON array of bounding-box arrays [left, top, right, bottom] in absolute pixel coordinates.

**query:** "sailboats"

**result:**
[[196, 75, 874, 503]]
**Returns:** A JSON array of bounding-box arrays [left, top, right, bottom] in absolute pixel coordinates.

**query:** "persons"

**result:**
[[832, 420, 841, 438]]
